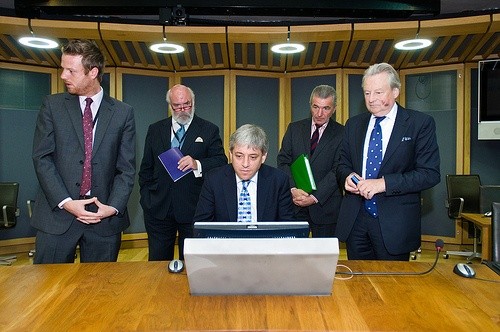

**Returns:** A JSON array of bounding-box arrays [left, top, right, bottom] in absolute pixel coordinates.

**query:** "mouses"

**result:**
[[453, 263, 476, 278], [168, 259, 184, 273]]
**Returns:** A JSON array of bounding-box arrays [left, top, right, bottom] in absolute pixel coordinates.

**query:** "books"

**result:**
[[158, 147, 193, 183], [291, 154, 317, 196]]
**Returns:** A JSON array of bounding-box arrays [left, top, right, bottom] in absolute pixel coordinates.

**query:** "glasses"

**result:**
[[170, 98, 192, 112]]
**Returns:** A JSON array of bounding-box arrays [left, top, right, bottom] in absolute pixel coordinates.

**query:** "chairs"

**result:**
[[445, 174, 492, 264], [0, 182, 19, 228]]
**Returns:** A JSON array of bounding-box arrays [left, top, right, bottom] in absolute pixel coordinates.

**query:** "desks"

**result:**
[[460, 213, 492, 262], [0, 261, 500, 332]]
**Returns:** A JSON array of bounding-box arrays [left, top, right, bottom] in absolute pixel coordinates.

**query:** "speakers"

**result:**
[[478, 59, 500, 140]]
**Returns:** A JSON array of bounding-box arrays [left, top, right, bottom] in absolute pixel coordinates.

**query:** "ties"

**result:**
[[365, 116, 386, 218], [237, 179, 252, 222], [171, 121, 186, 150], [80, 97, 93, 196], [310, 123, 323, 157]]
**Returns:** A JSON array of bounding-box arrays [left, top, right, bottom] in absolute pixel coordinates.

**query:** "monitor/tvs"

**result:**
[[192, 221, 309, 238]]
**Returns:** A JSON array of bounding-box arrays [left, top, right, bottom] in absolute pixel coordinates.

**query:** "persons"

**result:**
[[195, 124, 294, 222], [139, 82, 227, 262], [32, 38, 137, 265], [275, 84, 345, 238], [335, 63, 440, 261]]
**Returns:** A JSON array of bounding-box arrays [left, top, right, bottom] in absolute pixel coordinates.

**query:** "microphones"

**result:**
[[336, 238, 443, 276]]
